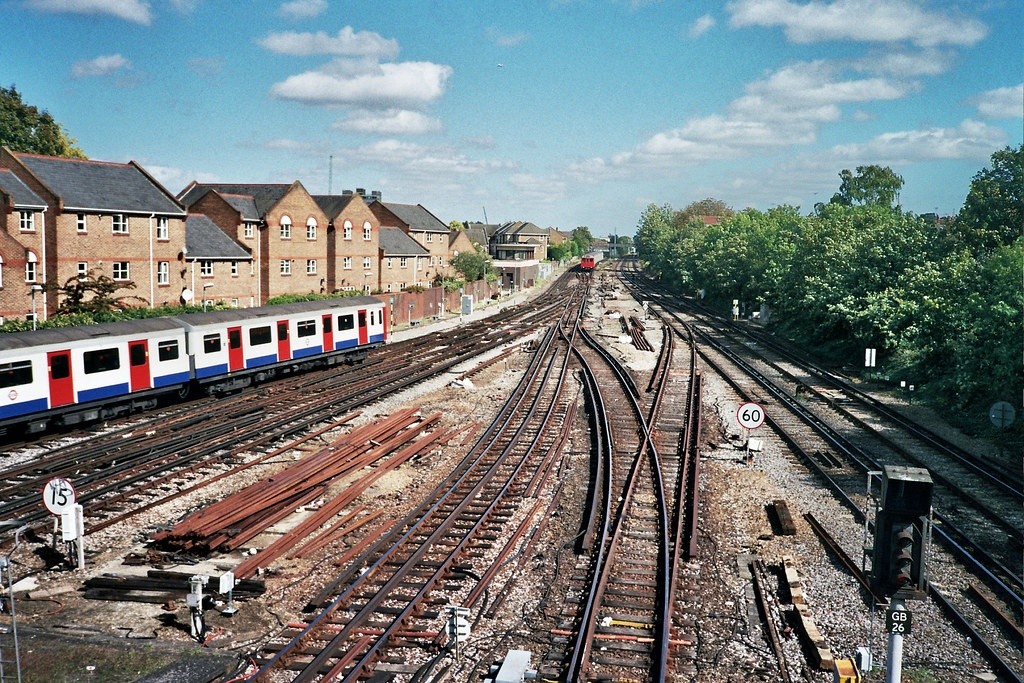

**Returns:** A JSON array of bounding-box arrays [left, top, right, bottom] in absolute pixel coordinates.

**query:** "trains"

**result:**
[[0, 296, 388, 444], [581, 251, 603, 271]]
